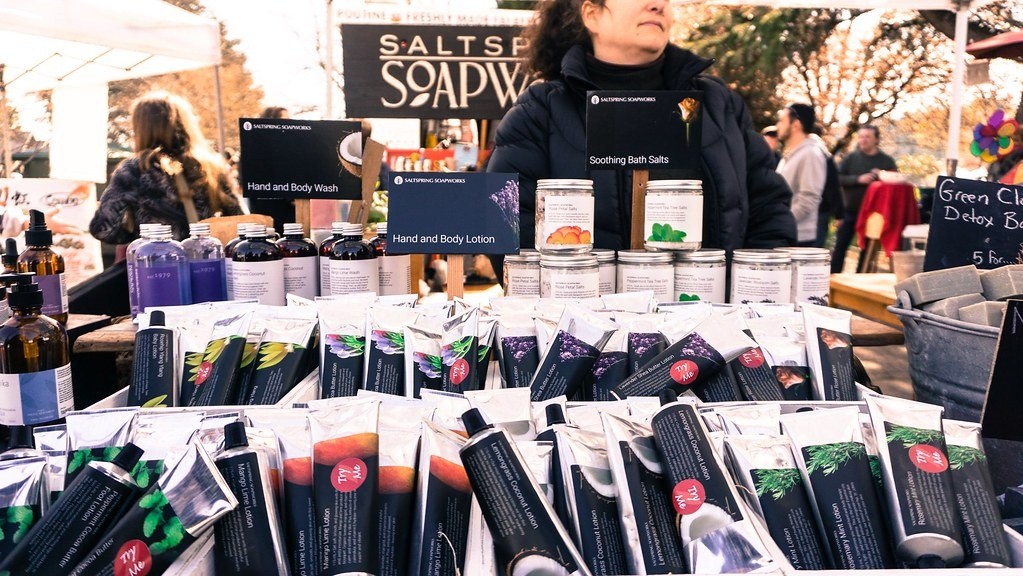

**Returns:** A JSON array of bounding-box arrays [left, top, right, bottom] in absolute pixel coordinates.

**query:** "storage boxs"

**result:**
[[70, 356, 1023, 576]]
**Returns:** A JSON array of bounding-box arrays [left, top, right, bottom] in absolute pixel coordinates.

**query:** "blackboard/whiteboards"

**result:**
[[923, 176, 1023, 272]]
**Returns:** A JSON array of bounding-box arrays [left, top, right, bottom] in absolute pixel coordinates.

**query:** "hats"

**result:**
[[781, 103, 815, 133]]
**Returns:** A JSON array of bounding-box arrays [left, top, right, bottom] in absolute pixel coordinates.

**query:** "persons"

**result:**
[[88, 94, 296, 246], [760, 103, 897, 274], [487, 0, 798, 288], [0, 206, 82, 237]]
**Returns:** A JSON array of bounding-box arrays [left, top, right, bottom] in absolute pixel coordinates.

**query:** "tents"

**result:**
[[0, 0, 226, 178]]
